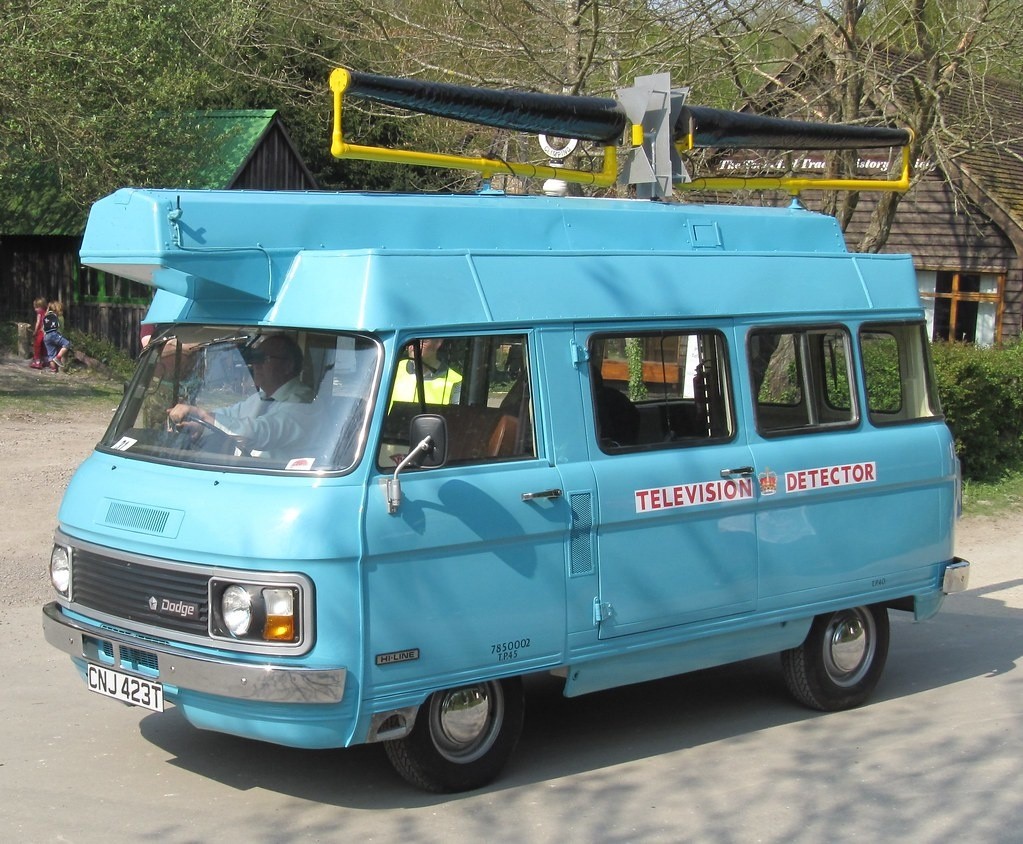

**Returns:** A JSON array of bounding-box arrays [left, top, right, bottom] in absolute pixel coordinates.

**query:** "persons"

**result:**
[[139, 288, 206, 432], [43, 300, 70, 371], [166, 333, 329, 464], [29, 296, 50, 369], [378, 338, 463, 467]]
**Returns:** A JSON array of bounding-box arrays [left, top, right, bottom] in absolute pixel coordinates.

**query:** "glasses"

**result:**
[[248, 353, 286, 364]]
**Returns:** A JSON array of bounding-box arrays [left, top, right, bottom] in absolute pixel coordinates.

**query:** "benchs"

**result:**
[[330, 398, 518, 462]]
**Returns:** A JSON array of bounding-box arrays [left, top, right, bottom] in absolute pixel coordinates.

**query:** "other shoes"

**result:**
[[29, 361, 43, 369], [41, 362, 50, 367], [52, 357, 66, 367], [48, 364, 58, 372]]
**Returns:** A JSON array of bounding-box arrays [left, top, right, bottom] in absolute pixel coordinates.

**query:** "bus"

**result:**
[[36, 190, 975, 799]]
